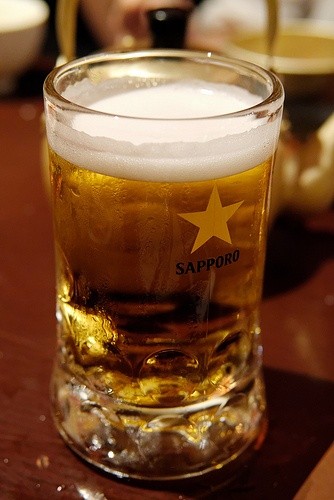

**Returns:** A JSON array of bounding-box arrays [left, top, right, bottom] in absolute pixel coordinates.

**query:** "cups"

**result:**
[[43, 49, 285, 480]]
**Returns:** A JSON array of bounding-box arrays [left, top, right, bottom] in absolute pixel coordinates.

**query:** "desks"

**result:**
[[0, 99, 334, 500]]
